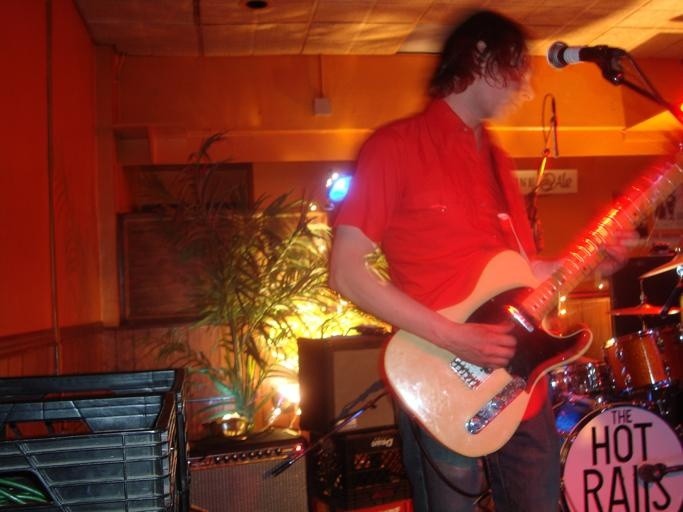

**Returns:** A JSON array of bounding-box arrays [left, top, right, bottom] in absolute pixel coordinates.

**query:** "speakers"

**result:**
[[298, 333, 396, 433], [185, 430, 308, 512]]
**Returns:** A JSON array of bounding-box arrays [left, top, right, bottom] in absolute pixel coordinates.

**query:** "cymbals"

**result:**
[[607, 290, 680, 317], [638, 247, 683, 277]]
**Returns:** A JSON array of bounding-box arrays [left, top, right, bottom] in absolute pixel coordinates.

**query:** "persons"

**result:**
[[327, 9, 628, 512]]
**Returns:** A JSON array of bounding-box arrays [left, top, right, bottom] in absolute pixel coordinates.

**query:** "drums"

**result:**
[[550, 355, 605, 399], [553, 390, 681, 511], [604, 324, 681, 403]]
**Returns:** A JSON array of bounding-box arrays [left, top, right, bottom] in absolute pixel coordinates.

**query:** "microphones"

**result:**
[[550, 96, 562, 157], [546, 41, 622, 70]]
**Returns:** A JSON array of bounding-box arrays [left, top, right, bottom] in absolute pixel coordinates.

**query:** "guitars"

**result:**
[[382, 135, 683, 459]]
